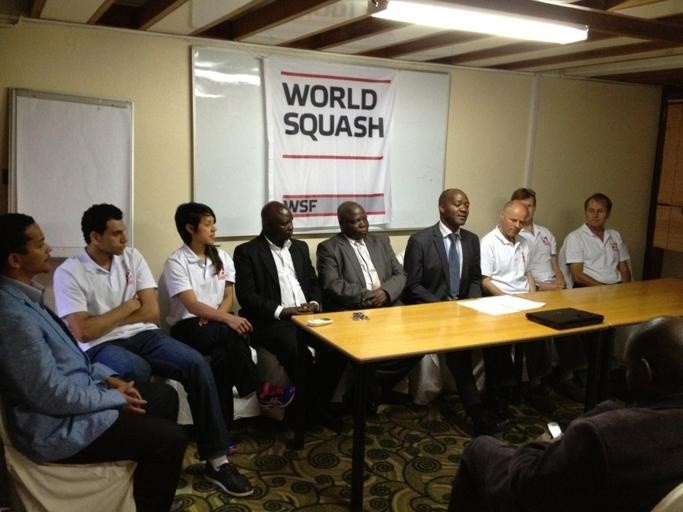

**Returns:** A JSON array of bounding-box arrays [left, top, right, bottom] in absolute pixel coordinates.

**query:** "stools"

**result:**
[[145, 338, 408, 424]]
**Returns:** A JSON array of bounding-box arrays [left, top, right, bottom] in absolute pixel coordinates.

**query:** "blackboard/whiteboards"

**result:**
[[189, 46, 451, 238], [6, 88, 134, 261]]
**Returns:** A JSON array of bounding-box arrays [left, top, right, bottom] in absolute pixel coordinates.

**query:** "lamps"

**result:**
[[364, 0, 591, 46]]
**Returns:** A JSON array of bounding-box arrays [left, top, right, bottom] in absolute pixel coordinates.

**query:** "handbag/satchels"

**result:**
[[255, 381, 296, 409]]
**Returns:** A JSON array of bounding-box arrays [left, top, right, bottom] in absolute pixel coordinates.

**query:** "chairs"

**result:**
[[651, 482, 683, 512], [0, 403, 137, 511], [395, 250, 484, 404]]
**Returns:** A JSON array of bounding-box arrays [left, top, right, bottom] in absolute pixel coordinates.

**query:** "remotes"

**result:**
[[307, 316, 333, 326]]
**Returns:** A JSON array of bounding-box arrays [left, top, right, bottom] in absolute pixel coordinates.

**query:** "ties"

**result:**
[[354, 240, 381, 291], [448, 233, 460, 298]]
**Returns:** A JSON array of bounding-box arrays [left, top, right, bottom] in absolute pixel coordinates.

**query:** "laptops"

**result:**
[[525, 307, 605, 330]]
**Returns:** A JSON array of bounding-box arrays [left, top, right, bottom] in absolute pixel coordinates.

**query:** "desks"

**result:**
[[291, 275, 682, 510]]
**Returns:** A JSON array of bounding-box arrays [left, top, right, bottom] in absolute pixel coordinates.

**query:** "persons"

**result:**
[[317, 202, 424, 412], [233, 200, 344, 424], [480, 188, 629, 390], [53, 203, 253, 496], [446, 312, 683, 511], [402, 189, 511, 427], [0, 212, 188, 512], [162, 203, 296, 455]]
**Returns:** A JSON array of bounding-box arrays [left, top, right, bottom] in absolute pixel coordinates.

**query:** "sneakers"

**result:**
[[525, 383, 557, 413], [202, 459, 254, 497], [553, 373, 586, 403]]
